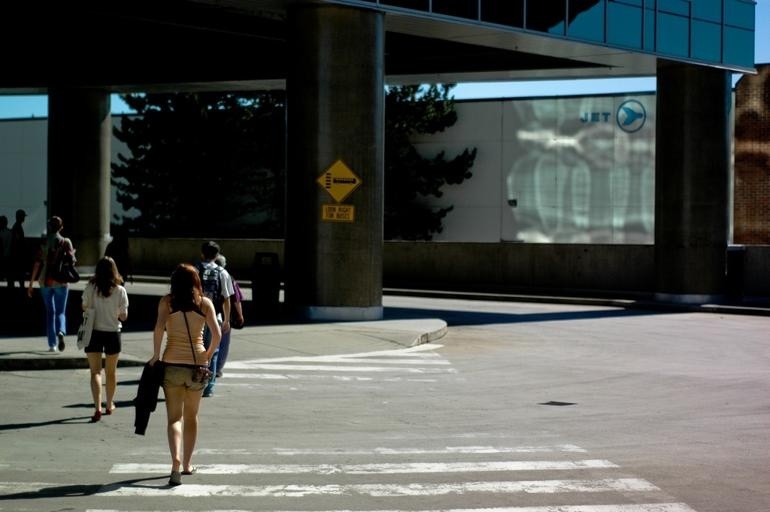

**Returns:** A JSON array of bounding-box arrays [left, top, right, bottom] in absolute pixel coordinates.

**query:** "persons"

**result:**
[[104, 228, 130, 287], [28, 216, 81, 352], [147, 261, 224, 486], [0, 215, 12, 281], [6, 208, 28, 292], [77, 256, 130, 421], [214, 254, 245, 377], [192, 240, 236, 397]]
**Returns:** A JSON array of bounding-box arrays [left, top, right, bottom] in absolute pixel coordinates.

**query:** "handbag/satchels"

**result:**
[[77, 308, 96, 350], [192, 367, 214, 384], [47, 261, 80, 283]]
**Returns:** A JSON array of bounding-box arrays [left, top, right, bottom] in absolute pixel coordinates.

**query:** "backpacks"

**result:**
[[199, 269, 221, 305]]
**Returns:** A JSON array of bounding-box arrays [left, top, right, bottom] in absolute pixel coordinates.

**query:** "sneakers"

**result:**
[[49, 332, 66, 352], [203, 391, 214, 397]]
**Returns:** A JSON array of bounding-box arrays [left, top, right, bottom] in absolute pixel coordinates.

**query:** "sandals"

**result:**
[[91, 407, 114, 422], [169, 466, 196, 485]]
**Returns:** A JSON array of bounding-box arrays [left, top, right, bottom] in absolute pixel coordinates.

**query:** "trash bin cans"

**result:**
[[726, 244, 770, 309], [252, 251, 279, 313]]
